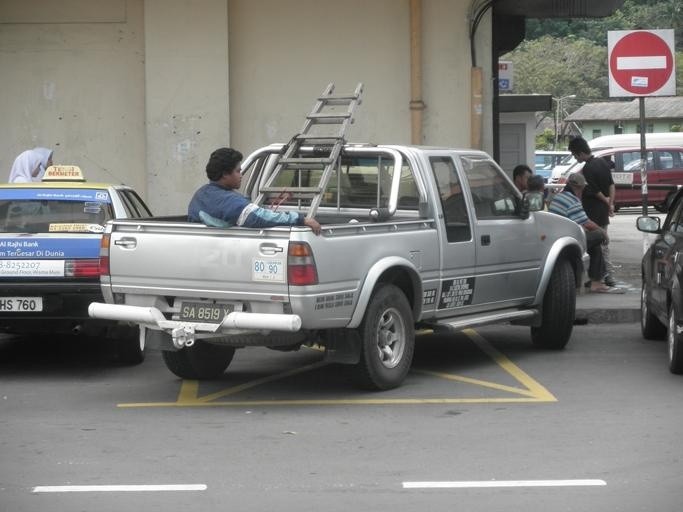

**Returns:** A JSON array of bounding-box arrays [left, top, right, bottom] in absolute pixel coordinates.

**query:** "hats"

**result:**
[[567, 173, 589, 187]]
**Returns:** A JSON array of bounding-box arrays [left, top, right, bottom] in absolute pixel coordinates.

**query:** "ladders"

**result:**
[[252, 81, 364, 219]]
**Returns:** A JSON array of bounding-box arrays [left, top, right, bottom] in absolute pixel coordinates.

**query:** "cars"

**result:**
[[1, 162, 161, 373], [630, 185, 681, 379]]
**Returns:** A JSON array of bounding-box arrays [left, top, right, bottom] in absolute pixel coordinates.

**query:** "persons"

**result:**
[[186, 146, 322, 237], [32, 145, 62, 214], [512, 165, 532, 192], [566, 137, 617, 287], [547, 172, 612, 295], [521, 175, 547, 214], [7, 149, 47, 220]]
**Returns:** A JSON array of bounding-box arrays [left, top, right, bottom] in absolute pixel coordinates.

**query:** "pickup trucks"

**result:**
[[88, 130, 599, 401]]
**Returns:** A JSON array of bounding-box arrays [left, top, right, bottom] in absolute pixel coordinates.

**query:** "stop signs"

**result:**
[[604, 29, 677, 98]]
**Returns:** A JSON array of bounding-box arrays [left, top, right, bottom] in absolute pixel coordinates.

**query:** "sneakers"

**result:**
[[583, 273, 620, 293]]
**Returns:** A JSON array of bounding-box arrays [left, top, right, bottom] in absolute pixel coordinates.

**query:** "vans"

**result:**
[[529, 127, 681, 215]]
